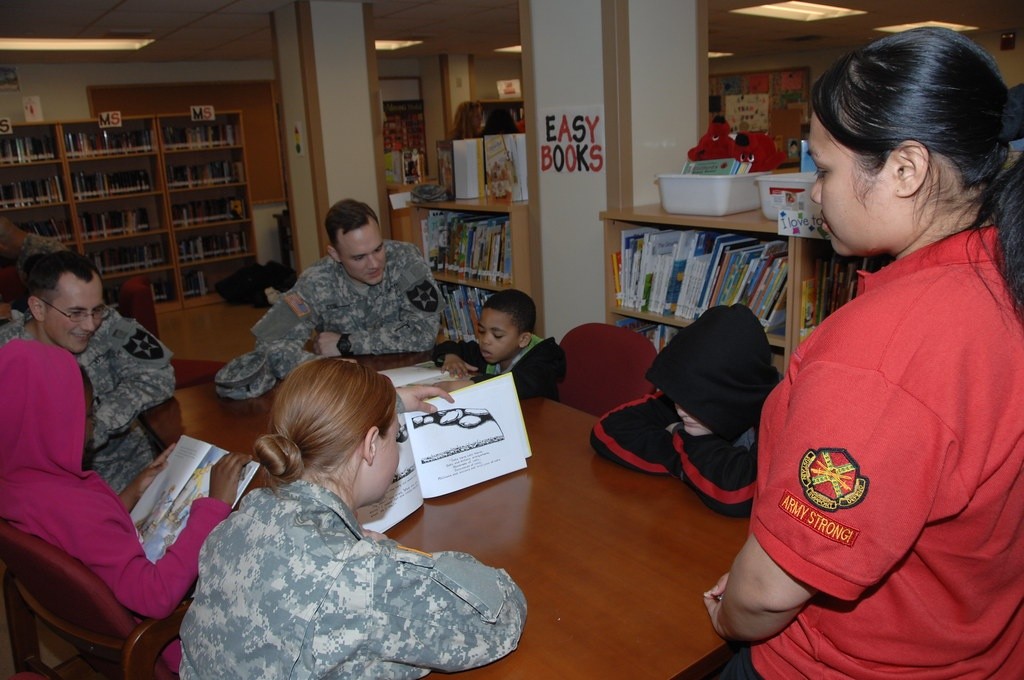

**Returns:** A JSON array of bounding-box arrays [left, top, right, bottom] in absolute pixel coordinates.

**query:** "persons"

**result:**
[[252, 198, 449, 357], [0, 251, 176, 496], [589, 304, 781, 518], [0, 338, 254, 673], [431, 289, 567, 402], [702, 26, 1024, 680], [178, 357, 528, 680], [0, 217, 71, 284]]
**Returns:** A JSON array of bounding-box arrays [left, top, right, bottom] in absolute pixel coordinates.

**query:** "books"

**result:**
[[182, 269, 208, 300], [354, 371, 532, 534], [611, 228, 869, 357], [434, 288, 496, 347], [0, 168, 171, 306], [63, 130, 151, 159], [160, 120, 247, 264], [0, 135, 55, 166], [129, 434, 260, 565], [373, 365, 475, 388], [420, 210, 511, 287]]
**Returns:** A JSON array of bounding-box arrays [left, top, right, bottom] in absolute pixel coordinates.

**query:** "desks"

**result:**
[[136, 351, 752, 680]]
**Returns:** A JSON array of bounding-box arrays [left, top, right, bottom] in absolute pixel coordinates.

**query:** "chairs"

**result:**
[[0, 517, 191, 680], [557, 322, 658, 418]]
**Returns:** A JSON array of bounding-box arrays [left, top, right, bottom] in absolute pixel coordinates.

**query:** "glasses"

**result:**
[[34, 295, 111, 323]]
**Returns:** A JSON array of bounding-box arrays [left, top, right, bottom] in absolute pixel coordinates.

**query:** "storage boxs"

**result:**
[[453, 133, 528, 201], [755, 172, 824, 221], [653, 170, 773, 217]]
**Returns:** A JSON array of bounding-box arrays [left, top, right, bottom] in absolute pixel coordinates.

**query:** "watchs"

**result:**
[[336, 334, 352, 356]]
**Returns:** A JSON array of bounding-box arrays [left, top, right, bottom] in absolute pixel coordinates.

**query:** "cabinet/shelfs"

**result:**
[[599, 203, 896, 376], [0, 109, 259, 314], [406, 202, 531, 346]]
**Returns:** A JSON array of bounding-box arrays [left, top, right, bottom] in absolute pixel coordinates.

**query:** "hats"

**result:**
[[410, 184, 448, 203], [213, 349, 277, 401]]
